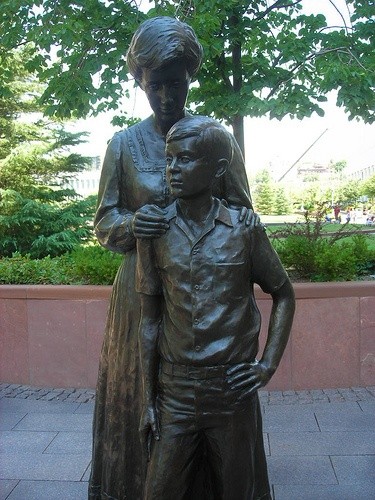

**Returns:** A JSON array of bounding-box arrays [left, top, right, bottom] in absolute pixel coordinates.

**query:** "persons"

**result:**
[[346, 211, 351, 223], [326, 214, 333, 224], [136, 114, 295, 500], [334, 206, 340, 219], [88, 16, 267, 500]]
